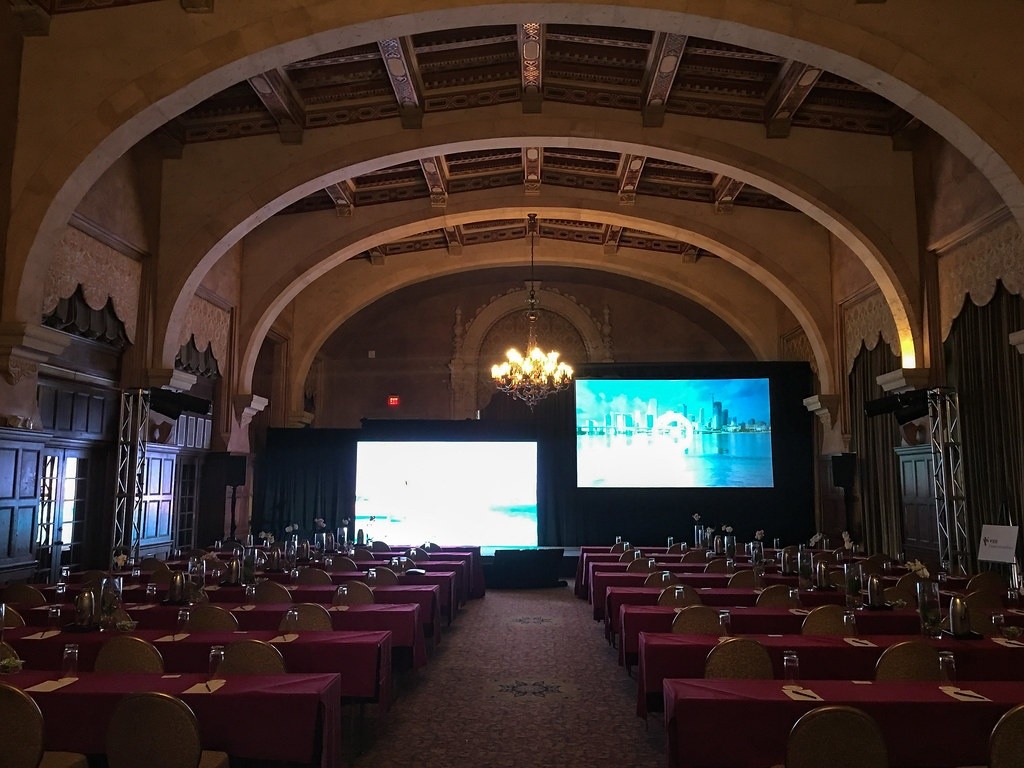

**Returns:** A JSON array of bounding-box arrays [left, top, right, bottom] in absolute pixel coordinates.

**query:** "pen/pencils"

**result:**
[[40, 632, 45, 638], [1016, 610, 1024, 613], [239, 606, 246, 611], [290, 586, 293, 590], [792, 690, 816, 699], [852, 639, 869, 645], [172, 634, 174, 641], [1004, 640, 1024, 646], [795, 609, 808, 614], [334, 606, 339, 612], [953, 689, 987, 700], [205, 683, 212, 692], [283, 634, 287, 641]]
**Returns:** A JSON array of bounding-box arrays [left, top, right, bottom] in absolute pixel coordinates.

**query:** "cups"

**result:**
[[939, 650, 958, 693], [782, 650, 803, 691], [392, 557, 399, 567], [648, 558, 656, 568], [207, 645, 225, 682], [662, 522, 1024, 640], [623, 542, 630, 552], [1, 529, 378, 632], [615, 536, 622, 545], [425, 541, 431, 552], [410, 547, 417, 560], [61, 644, 79, 678], [633, 549, 641, 560]]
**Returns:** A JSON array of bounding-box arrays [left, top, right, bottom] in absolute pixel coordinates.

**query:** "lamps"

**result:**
[[490, 212, 575, 416]]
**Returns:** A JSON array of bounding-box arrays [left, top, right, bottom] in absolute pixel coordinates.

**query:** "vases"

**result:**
[[724, 536, 736, 559], [262, 540, 269, 548], [292, 535, 298, 545], [188, 556, 205, 585], [314, 533, 326, 552], [750, 541, 764, 562], [915, 582, 942, 640], [694, 525, 703, 548], [337, 527, 348, 546]]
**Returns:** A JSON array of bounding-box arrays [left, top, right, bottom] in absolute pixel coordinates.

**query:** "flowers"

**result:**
[[903, 558, 929, 579], [693, 514, 853, 549], [201, 552, 218, 561], [258, 517, 351, 543], [113, 552, 127, 570]]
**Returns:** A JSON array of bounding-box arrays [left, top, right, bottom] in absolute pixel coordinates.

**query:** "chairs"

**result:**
[[576, 542, 1024, 768], [0, 541, 444, 768]]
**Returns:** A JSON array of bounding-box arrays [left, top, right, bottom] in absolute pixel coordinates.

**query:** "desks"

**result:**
[[636, 632, 1019, 717], [586, 562, 844, 600], [606, 586, 846, 646], [0, 547, 483, 768], [663, 679, 1024, 763], [583, 551, 759, 590], [620, 604, 950, 677], [580, 545, 702, 551], [590, 570, 800, 639]]
